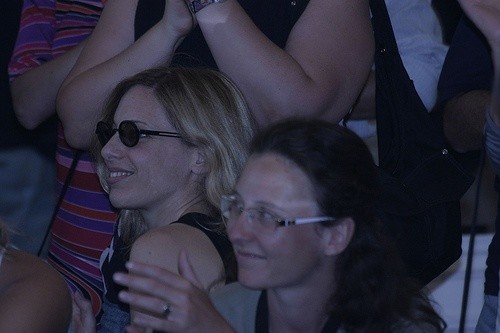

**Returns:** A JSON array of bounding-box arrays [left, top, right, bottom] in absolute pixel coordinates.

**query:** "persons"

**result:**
[[0, 0, 500, 333], [8, 0, 160, 333]]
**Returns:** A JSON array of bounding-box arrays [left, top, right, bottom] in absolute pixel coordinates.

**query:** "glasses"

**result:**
[[220, 195, 335, 233], [95, 122, 181, 147]]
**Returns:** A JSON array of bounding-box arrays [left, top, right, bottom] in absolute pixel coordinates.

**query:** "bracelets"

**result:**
[[186, 0, 231, 14]]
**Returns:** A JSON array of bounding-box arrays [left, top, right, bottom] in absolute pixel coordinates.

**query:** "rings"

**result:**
[[161, 302, 172, 318]]
